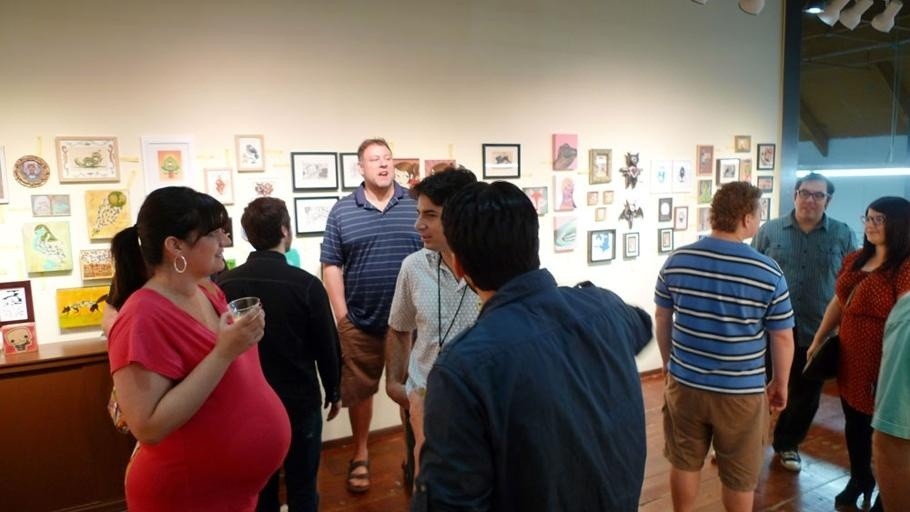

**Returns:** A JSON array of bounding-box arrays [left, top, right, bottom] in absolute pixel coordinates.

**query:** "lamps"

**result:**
[[694, 0, 903, 34]]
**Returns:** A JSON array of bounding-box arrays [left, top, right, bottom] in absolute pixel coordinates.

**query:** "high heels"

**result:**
[[835, 473, 875, 505]]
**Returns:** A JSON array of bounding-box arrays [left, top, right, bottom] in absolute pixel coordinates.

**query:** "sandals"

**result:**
[[347, 454, 369, 493]]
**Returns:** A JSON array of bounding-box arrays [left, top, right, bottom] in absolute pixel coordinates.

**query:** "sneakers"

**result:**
[[777, 449, 800, 471]]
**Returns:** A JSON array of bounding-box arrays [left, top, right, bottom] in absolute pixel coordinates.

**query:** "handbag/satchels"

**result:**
[[802, 334, 842, 378]]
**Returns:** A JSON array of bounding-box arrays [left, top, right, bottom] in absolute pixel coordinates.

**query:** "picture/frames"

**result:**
[[295, 195, 341, 237], [13, 155, 72, 216], [524, 186, 549, 216], [2, 280, 37, 327], [588, 149, 613, 184], [291, 152, 337, 190], [658, 228, 674, 254], [673, 207, 689, 230], [659, 198, 672, 222], [205, 169, 238, 207], [394, 159, 419, 188], [79, 249, 115, 281], [57, 285, 112, 331], [555, 177, 578, 210], [482, 144, 521, 180], [698, 136, 775, 234], [22, 222, 70, 275], [554, 216, 577, 253], [236, 139, 265, 173], [138, 139, 196, 197], [55, 139, 122, 182], [552, 137, 576, 170], [339, 154, 362, 191], [587, 190, 614, 221], [587, 228, 616, 263], [654, 160, 691, 194], [623, 234, 639, 257], [88, 191, 132, 240], [423, 161, 457, 175], [1, 150, 10, 205]]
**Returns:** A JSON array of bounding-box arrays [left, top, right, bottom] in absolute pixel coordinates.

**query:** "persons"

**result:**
[[653, 179, 798, 511], [870, 291, 908, 509], [748, 172, 860, 470], [382, 164, 483, 409], [212, 196, 343, 512], [317, 137, 424, 495], [407, 180, 653, 512], [105, 187, 292, 511], [802, 195, 908, 510]]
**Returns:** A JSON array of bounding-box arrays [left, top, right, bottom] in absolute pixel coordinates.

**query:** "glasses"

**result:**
[[796, 190, 829, 201], [860, 215, 885, 224]]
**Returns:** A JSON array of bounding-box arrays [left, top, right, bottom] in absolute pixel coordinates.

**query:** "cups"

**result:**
[[226, 296, 261, 323]]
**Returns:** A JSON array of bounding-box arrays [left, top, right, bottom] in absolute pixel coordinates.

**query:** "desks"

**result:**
[[0, 337, 134, 512]]
